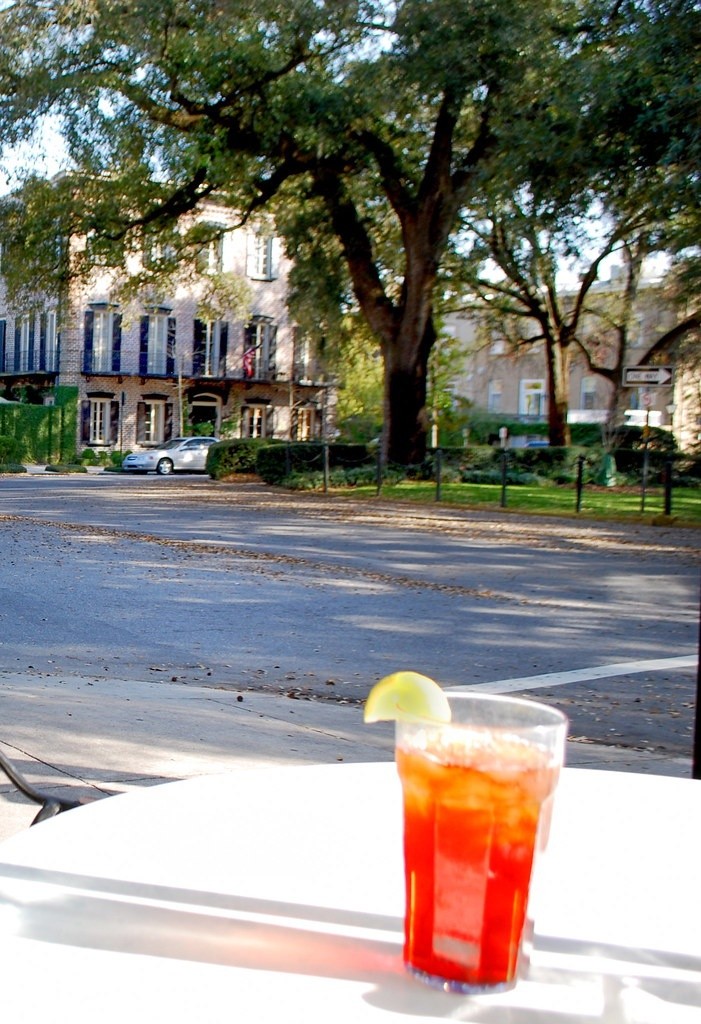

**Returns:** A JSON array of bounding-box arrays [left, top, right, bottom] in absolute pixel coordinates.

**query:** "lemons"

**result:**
[[363, 671, 452, 724]]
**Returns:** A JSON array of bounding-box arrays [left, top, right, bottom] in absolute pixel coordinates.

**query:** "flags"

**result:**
[[242, 347, 255, 377]]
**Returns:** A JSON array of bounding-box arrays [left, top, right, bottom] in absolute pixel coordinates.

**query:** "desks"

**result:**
[[0, 759, 701, 1024]]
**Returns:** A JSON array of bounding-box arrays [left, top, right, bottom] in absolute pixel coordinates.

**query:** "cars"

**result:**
[[120, 436, 227, 475]]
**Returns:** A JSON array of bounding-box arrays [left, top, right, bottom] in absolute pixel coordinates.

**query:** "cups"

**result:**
[[394, 693, 570, 996]]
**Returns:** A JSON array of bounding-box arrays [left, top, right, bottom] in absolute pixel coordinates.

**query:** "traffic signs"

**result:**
[[622, 364, 678, 387]]
